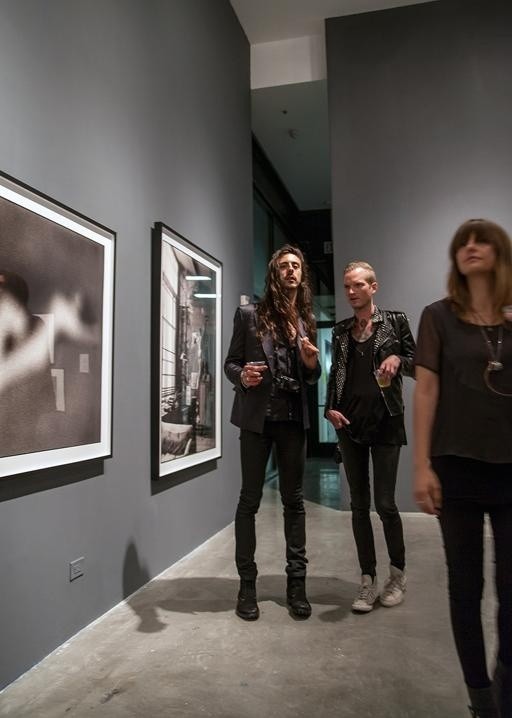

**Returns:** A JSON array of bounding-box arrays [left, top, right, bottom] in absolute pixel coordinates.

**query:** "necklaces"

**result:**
[[472, 311, 504, 371], [354, 316, 372, 343]]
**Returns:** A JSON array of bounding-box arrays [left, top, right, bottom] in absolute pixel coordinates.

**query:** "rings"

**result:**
[[244, 371, 247, 377], [417, 500, 424, 504], [247, 377, 249, 381]]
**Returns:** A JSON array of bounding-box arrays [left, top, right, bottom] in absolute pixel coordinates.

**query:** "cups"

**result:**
[[375, 369, 392, 388], [247, 361, 265, 376]]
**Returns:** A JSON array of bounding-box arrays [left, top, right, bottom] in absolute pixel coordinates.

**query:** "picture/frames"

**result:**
[[151, 219, 223, 480], [0, 170, 119, 483]]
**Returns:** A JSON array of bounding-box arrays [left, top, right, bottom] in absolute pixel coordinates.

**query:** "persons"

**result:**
[[324, 259, 416, 612], [411, 219, 512, 718], [223, 245, 321, 620]]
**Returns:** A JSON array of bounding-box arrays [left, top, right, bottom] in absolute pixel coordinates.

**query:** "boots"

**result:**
[[467, 659, 512, 717], [352, 574, 381, 612], [380, 565, 407, 606], [286, 577, 312, 615], [237, 581, 259, 620]]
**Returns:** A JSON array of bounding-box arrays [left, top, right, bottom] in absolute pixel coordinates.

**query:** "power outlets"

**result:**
[[69, 557, 85, 581]]
